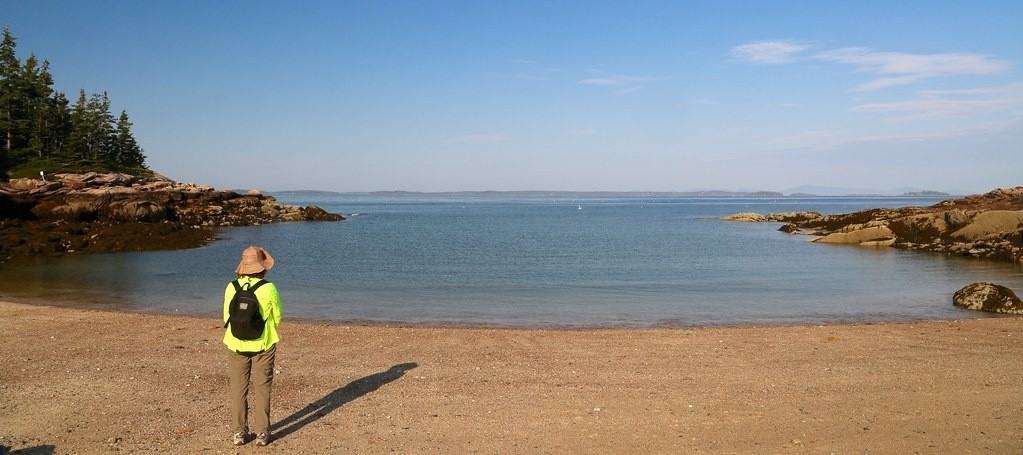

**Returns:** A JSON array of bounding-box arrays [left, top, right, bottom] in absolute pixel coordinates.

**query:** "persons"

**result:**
[[222, 246, 281, 445]]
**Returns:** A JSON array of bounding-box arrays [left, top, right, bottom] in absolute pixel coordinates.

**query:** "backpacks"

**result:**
[[224, 279, 272, 339]]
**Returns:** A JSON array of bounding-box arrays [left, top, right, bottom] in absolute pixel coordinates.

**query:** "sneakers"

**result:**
[[255, 433, 273, 446], [233, 432, 251, 445]]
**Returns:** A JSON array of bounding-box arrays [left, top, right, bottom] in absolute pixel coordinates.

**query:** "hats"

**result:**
[[235, 246, 274, 274]]
